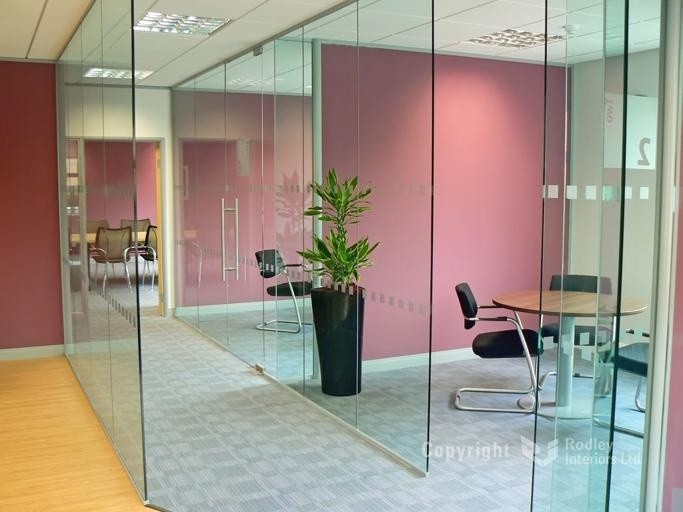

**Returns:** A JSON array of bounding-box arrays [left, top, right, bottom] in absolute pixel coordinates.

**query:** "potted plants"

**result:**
[[295, 167, 381, 396]]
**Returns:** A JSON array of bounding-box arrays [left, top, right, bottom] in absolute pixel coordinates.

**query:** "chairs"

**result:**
[[454, 274, 648, 437], [256, 249, 313, 334], [87, 218, 157, 294]]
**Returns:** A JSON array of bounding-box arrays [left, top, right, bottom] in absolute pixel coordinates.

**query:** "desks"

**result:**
[[69, 228, 158, 291]]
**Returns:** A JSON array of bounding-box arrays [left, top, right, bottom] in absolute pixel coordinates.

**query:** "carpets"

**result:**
[[70, 263, 647, 512]]
